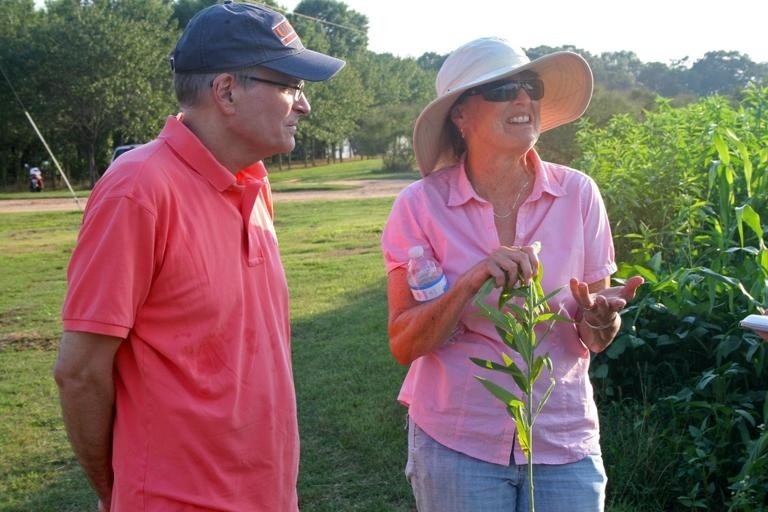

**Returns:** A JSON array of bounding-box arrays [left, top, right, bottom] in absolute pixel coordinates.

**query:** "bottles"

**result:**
[[403, 245, 466, 348]]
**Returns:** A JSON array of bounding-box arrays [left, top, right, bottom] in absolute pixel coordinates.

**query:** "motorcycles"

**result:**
[[23, 159, 51, 192]]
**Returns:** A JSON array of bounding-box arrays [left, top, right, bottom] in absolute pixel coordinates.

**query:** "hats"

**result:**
[[413, 37, 595, 178], [170, 2, 346, 82]]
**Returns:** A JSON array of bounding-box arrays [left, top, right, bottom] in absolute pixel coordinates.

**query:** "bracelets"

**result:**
[[583, 312, 617, 331]]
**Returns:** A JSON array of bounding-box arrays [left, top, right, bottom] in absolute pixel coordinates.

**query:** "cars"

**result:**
[[109, 144, 145, 164]]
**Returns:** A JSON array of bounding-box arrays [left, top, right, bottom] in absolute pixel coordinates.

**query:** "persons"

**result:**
[[52, 1, 347, 512], [382, 36, 646, 512]]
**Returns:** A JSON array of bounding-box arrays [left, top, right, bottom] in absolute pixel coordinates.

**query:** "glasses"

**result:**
[[456, 78, 543, 103], [210, 73, 305, 101]]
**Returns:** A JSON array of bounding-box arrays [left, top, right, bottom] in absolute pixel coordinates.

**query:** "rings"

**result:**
[[583, 306, 595, 311]]
